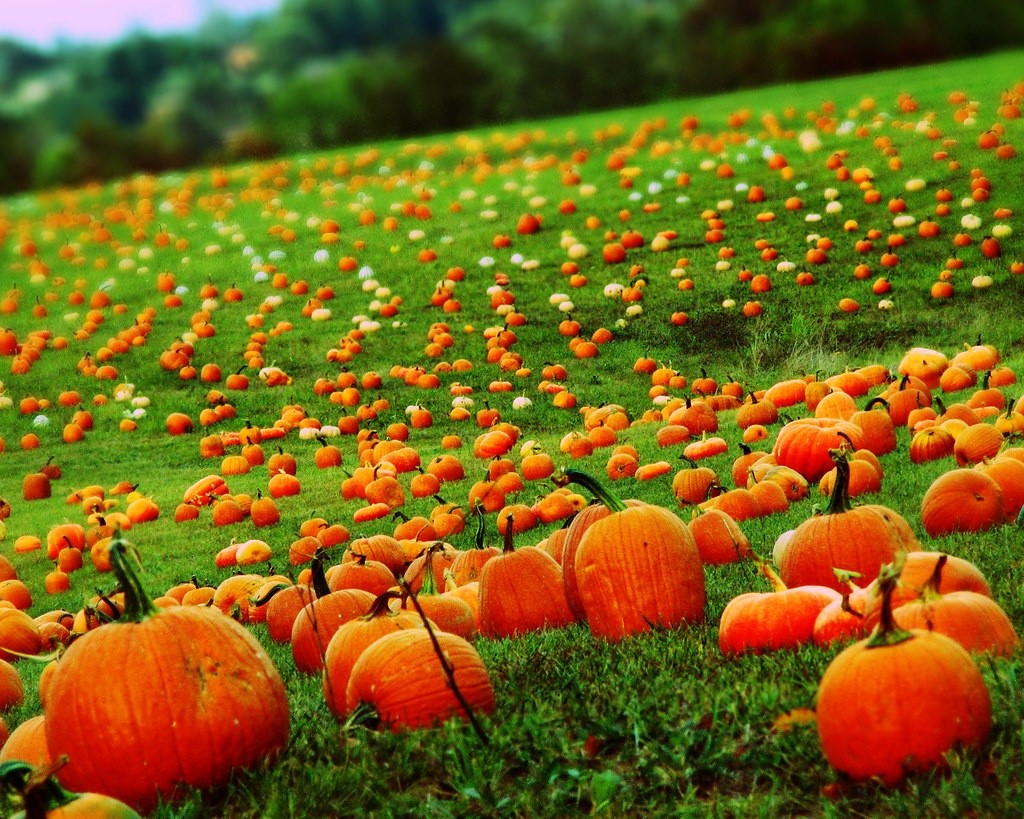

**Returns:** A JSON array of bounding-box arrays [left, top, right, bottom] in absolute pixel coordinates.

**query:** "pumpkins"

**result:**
[[0, 80, 1024, 819]]
